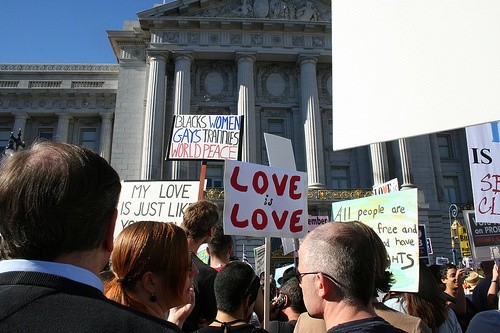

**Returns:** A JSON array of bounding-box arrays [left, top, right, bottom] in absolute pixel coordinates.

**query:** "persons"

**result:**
[[270, 219, 500, 333], [190, 260, 269, 333], [0, 127, 265, 333]]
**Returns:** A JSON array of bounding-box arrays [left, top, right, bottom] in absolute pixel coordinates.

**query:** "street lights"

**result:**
[[448, 204, 461, 266]]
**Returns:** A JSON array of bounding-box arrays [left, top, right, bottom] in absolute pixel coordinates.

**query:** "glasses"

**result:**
[[294, 268, 342, 287], [229, 260, 257, 295], [187, 252, 194, 271]]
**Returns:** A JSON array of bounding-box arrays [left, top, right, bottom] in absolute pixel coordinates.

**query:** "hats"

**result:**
[[278, 267, 296, 286], [464, 272, 479, 291]]
